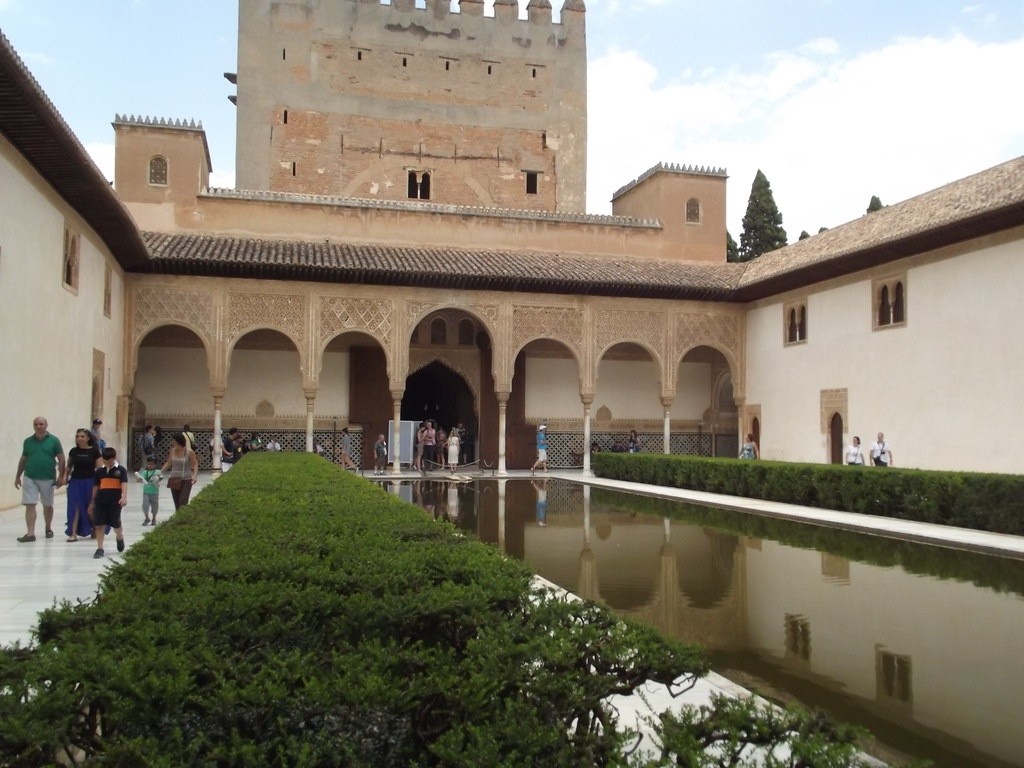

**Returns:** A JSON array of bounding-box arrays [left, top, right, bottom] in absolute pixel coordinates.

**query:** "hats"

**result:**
[[94, 419, 102, 424], [540, 425, 546, 431]]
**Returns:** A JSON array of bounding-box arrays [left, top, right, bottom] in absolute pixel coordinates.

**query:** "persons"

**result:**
[[87, 447, 129, 558], [869, 432, 895, 467], [341, 427, 359, 473], [627, 429, 642, 452], [406, 479, 551, 526], [412, 418, 472, 477], [136, 423, 281, 482], [612, 442, 625, 452], [590, 443, 601, 453], [736, 434, 760, 459], [313, 436, 324, 457], [159, 433, 198, 511], [61, 428, 111, 542], [373, 434, 388, 475], [136, 455, 164, 526], [91, 418, 102, 447], [846, 436, 867, 466], [14, 415, 65, 542], [530, 425, 551, 474]]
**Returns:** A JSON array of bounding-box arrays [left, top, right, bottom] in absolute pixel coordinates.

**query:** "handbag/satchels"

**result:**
[[191, 440, 196, 452], [167, 477, 182, 490]]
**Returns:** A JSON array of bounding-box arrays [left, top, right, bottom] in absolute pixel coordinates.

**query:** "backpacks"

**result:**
[[144, 439, 154, 455]]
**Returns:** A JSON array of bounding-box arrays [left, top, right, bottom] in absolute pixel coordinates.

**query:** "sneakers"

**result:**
[[95, 549, 104, 558], [115, 537, 125, 552]]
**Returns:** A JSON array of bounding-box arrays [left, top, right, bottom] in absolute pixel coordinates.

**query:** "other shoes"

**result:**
[[152, 519, 156, 524], [18, 535, 36, 542], [354, 466, 550, 475], [142, 519, 150, 525], [46, 531, 54, 537]]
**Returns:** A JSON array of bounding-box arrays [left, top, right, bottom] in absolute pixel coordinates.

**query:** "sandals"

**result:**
[[67, 534, 79, 542]]
